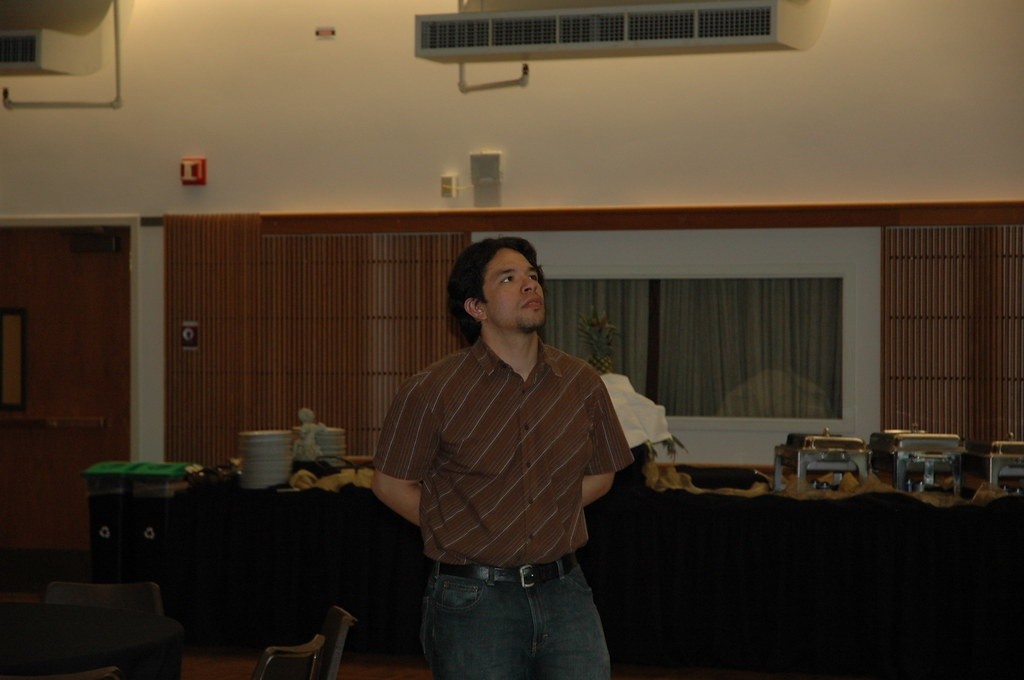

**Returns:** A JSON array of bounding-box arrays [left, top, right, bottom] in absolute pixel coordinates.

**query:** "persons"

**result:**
[[368, 238, 633, 680]]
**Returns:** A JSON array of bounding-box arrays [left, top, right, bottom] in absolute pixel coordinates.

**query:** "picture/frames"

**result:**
[[0, 305, 28, 415]]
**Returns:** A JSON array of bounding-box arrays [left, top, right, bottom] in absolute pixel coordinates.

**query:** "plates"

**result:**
[[238, 429, 293, 490], [292, 425, 346, 467]]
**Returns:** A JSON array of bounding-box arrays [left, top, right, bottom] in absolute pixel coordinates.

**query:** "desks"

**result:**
[[0, 599, 188, 680], [84, 470, 1023, 680]]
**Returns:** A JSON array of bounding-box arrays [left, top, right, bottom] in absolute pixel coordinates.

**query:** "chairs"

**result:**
[[43, 580, 164, 615], [250, 605, 360, 680]]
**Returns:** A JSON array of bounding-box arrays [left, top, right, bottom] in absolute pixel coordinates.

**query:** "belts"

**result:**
[[423, 554, 579, 588]]
